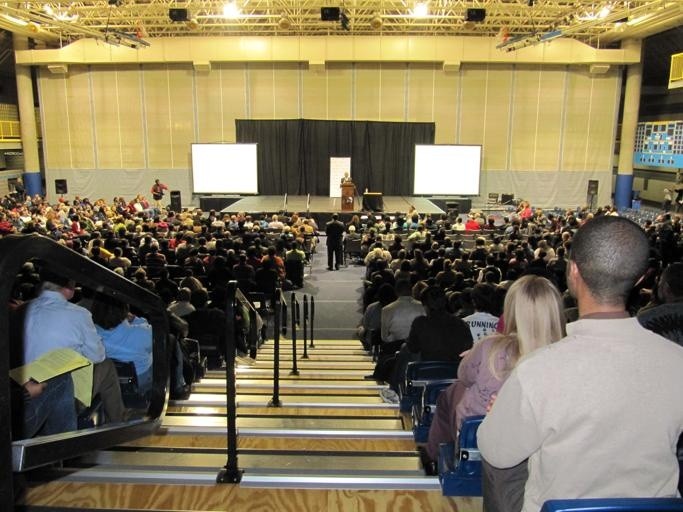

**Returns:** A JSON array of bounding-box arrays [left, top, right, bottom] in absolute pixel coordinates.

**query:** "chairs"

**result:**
[[77, 235, 227, 418]]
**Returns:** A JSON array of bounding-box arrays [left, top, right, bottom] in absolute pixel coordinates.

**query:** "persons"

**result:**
[[474, 215, 683, 512], [2, 173, 683, 439]]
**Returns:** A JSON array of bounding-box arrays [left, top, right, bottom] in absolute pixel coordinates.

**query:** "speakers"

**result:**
[[170, 190, 180, 210], [446, 202, 458, 221]]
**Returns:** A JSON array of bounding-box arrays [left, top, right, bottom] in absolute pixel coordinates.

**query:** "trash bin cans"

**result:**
[[170, 191, 181, 213], [501, 193, 514, 205]]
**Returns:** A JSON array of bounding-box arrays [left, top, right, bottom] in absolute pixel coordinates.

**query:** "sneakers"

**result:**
[[380, 388, 400, 403], [325, 264, 339, 270]]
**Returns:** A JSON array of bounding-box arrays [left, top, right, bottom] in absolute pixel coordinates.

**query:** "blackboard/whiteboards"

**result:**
[[329, 157, 351, 197]]
